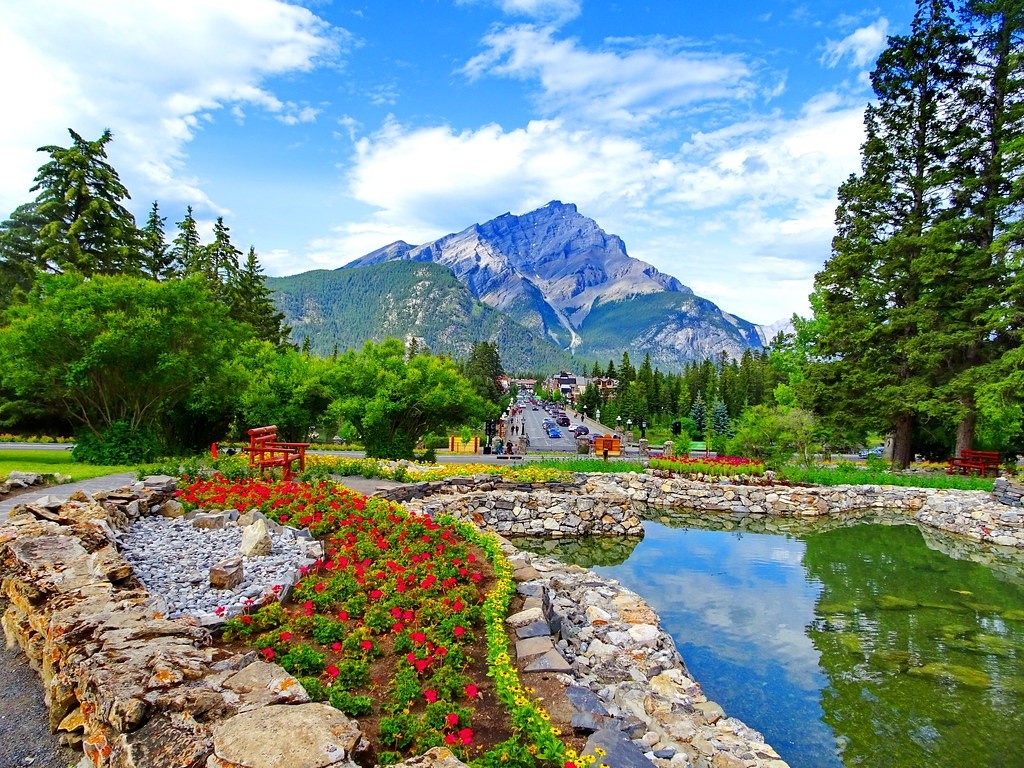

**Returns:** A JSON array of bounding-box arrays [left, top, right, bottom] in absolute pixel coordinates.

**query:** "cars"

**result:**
[[516, 390, 603, 444], [859, 447, 885, 459]]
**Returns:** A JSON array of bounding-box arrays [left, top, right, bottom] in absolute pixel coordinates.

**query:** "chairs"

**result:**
[[244, 425, 310, 480]]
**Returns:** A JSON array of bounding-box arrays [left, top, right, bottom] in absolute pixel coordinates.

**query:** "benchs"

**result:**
[[948, 450, 999, 477]]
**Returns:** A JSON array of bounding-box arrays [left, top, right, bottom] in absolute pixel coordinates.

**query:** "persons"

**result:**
[[511, 425, 519, 435], [510, 409, 516, 425], [496, 439, 514, 455], [526, 433, 530, 444], [581, 414, 584, 422]]
